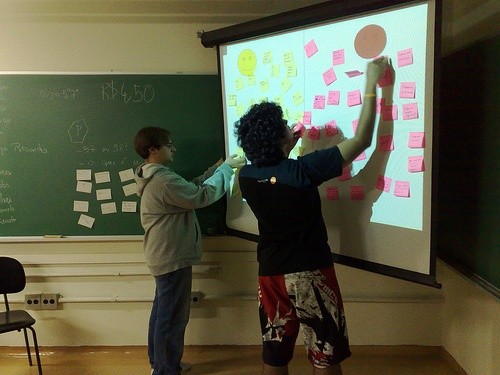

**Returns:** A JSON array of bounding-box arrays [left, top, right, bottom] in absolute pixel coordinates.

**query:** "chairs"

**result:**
[[0, 256, 43, 375]]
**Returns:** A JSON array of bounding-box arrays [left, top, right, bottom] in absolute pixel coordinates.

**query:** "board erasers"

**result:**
[[43, 234, 63, 238]]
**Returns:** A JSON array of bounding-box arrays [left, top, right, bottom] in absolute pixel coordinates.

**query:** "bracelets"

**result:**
[[364, 94, 376, 97]]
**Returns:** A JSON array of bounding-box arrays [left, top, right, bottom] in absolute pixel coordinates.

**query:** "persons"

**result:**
[[233, 56, 390, 375], [135, 127, 247, 375]]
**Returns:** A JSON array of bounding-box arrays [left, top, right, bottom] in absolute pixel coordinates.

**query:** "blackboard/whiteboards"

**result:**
[[0, 70, 229, 243], [432, 30, 500, 300]]
